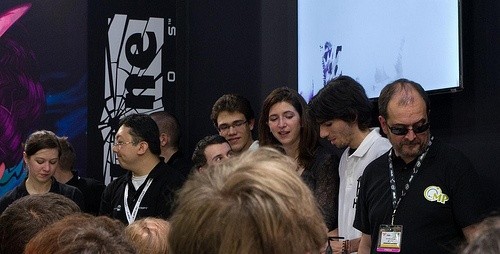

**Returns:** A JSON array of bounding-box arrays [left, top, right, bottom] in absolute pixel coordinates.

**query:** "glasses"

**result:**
[[112, 141, 140, 148], [218, 119, 249, 132], [386, 119, 429, 135]]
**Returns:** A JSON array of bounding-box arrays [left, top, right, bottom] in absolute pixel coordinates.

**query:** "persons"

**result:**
[[126, 217, 172, 254], [0, 193, 82, 254], [209, 94, 260, 154], [169, 147, 329, 254], [352, 79, 487, 254], [148, 111, 196, 175], [307, 75, 392, 254], [257, 86, 340, 243], [23, 212, 135, 254], [53, 136, 107, 216], [99, 113, 189, 227], [192, 134, 234, 175], [0, 130, 84, 216], [452, 211, 500, 254]]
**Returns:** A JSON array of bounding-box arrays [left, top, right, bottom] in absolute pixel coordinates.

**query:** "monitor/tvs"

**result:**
[[295, 0, 468, 103]]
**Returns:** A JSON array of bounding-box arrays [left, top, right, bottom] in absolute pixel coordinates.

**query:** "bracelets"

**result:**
[[341, 240, 351, 254]]
